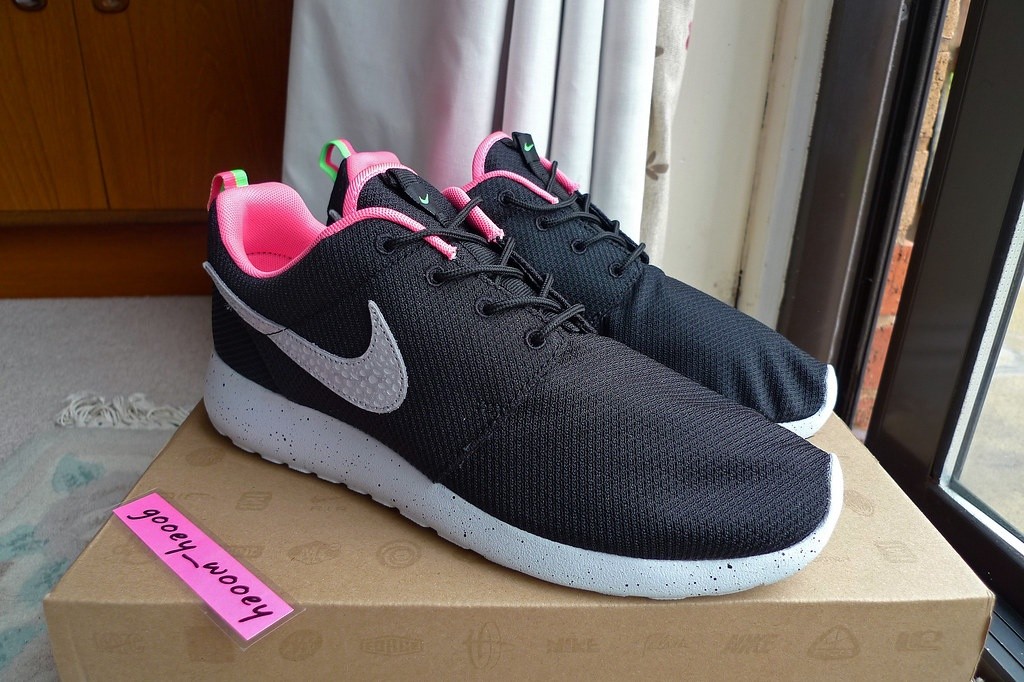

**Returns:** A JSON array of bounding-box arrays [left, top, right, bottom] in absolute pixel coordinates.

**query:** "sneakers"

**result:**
[[204, 140, 844, 597], [319, 130, 839, 439]]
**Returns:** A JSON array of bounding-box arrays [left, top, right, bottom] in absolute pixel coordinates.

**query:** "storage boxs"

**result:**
[[45, 398, 994, 682]]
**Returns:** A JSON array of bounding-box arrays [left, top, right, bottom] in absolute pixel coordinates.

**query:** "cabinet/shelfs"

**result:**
[[1, 0, 294, 298]]
